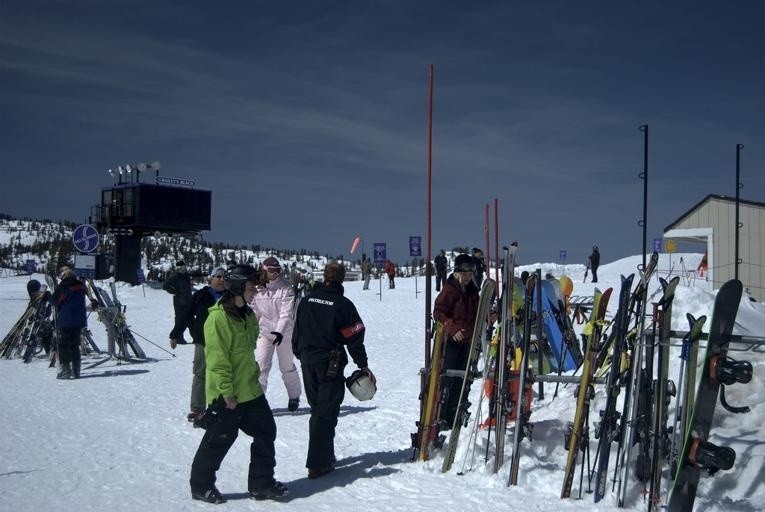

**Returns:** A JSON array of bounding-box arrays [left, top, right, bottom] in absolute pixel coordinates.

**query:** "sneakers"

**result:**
[[191, 487, 226, 504], [71, 368, 80, 377], [288, 397, 299, 410], [57, 370, 71, 378], [308, 460, 335, 479], [177, 338, 187, 344], [251, 481, 288, 498], [187, 409, 206, 422]]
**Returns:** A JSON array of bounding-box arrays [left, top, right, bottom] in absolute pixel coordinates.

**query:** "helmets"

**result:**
[[224, 264, 258, 296], [347, 371, 377, 402]]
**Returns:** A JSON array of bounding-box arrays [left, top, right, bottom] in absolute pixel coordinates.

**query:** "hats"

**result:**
[[454, 253, 477, 273], [60, 266, 71, 280], [263, 257, 281, 269], [176, 261, 185, 266]]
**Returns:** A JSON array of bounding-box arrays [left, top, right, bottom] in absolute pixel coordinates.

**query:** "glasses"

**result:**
[[214, 275, 222, 279], [263, 265, 280, 273]]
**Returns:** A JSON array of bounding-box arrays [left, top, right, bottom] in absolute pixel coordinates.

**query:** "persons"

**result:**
[[588, 245, 601, 284], [168, 267, 229, 420], [291, 262, 371, 480], [46, 264, 91, 381], [433, 249, 448, 291], [249, 257, 301, 412], [189, 264, 289, 503], [163, 263, 196, 344], [361, 258, 372, 290], [434, 254, 499, 421], [383, 259, 398, 290], [471, 246, 489, 290]]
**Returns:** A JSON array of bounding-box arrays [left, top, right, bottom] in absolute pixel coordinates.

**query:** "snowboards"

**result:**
[[515, 276, 584, 374], [665, 278, 743, 511]]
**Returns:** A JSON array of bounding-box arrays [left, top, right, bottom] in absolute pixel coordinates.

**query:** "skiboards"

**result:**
[[412, 241, 707, 512], [560, 285, 612, 500], [441, 277, 496, 475], [612, 250, 659, 508], [491, 241, 520, 474], [593, 273, 637, 503], [583, 258, 591, 284], [0, 277, 146, 368], [508, 275, 537, 486], [648, 275, 680, 511], [680, 313, 706, 463]]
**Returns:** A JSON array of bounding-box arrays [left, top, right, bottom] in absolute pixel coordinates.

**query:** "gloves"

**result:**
[[270, 332, 283, 346]]
